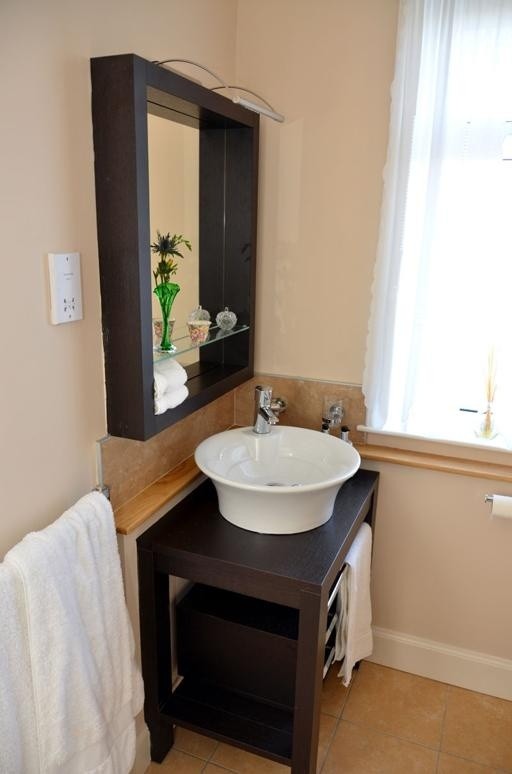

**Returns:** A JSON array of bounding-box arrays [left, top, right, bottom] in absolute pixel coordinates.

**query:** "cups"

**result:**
[[153, 319, 176, 337], [186, 321, 211, 342]]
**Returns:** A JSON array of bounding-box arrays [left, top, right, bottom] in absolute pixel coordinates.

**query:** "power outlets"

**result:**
[[47, 251, 83, 325]]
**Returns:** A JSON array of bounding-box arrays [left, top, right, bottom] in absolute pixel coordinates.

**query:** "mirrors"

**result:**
[[145, 99, 229, 383]]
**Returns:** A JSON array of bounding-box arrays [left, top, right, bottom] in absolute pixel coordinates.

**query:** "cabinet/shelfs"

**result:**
[[135, 467, 381, 774], [88, 52, 261, 442]]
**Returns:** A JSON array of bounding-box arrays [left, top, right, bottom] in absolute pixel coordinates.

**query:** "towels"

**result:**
[[0, 493, 147, 774], [152, 356, 191, 414], [338, 521, 374, 694]]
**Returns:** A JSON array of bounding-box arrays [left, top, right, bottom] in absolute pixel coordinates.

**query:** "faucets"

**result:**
[[253, 385, 280, 434]]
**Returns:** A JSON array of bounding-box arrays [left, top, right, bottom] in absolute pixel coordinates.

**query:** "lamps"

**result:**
[[152, 58, 285, 124]]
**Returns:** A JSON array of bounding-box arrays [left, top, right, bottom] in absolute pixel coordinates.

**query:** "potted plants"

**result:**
[[149, 228, 192, 353]]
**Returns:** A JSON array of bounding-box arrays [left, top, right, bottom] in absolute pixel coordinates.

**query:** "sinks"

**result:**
[[195, 425, 361, 535]]
